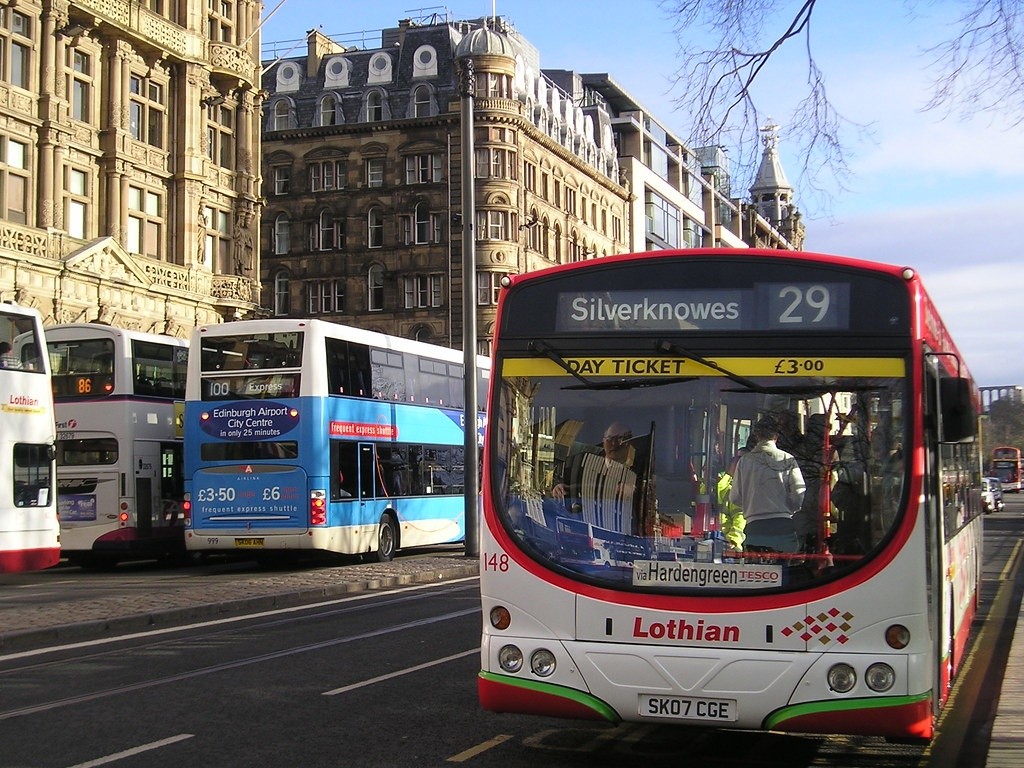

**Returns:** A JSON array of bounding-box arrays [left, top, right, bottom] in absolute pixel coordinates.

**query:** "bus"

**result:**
[[180, 320, 519, 566], [8, 322, 243, 572], [990, 445, 1024, 493], [478, 247, 984, 745], [0, 301, 63, 575]]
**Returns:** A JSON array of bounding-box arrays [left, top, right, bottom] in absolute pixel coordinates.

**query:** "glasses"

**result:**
[[602, 436, 628, 444]]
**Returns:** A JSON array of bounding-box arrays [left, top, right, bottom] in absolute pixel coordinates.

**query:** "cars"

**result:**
[[988, 476, 1005, 512], [979, 476, 996, 512]]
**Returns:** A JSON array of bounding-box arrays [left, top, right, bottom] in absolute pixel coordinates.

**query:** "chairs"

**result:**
[[248, 351, 296, 368], [136, 374, 173, 396]]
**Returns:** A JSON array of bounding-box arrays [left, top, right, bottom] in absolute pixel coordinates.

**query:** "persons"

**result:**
[[700, 411, 904, 565], [0, 342, 12, 368], [393, 470, 404, 495], [552, 421, 653, 536]]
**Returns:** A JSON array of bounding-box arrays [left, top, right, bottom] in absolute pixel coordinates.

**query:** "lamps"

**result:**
[[519, 220, 538, 231], [201, 96, 227, 106], [54, 25, 86, 37]]
